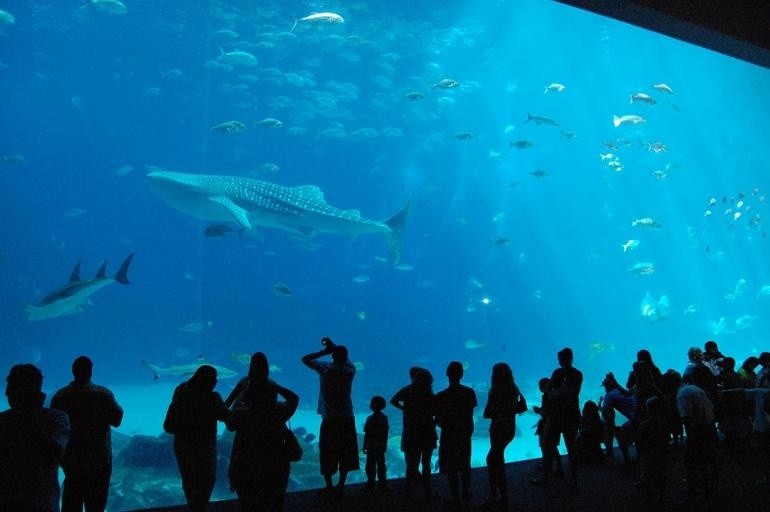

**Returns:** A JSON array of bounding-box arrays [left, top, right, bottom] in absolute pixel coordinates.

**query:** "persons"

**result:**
[[301, 333, 359, 488], [1, 358, 67, 510], [360, 386, 392, 487], [50, 352, 123, 512], [390, 335, 770, 478], [160, 361, 234, 512], [220, 351, 306, 512]]
